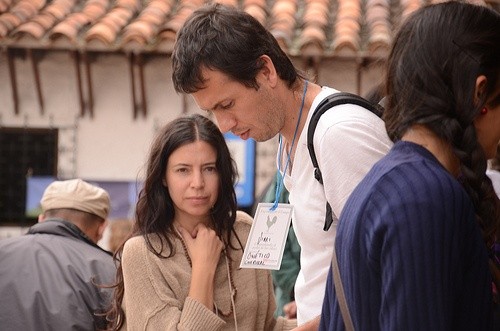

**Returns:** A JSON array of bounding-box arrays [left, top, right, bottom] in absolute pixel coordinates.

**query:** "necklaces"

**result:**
[[171, 222, 193, 267]]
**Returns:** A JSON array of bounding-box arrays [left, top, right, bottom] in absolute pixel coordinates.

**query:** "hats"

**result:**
[[41, 177, 110, 220]]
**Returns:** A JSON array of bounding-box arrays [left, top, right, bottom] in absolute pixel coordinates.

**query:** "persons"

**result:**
[[254, 171, 301, 317], [0, 178, 125, 331], [90, 113, 298, 331], [317, 0, 500, 331], [169, 0, 394, 331]]
